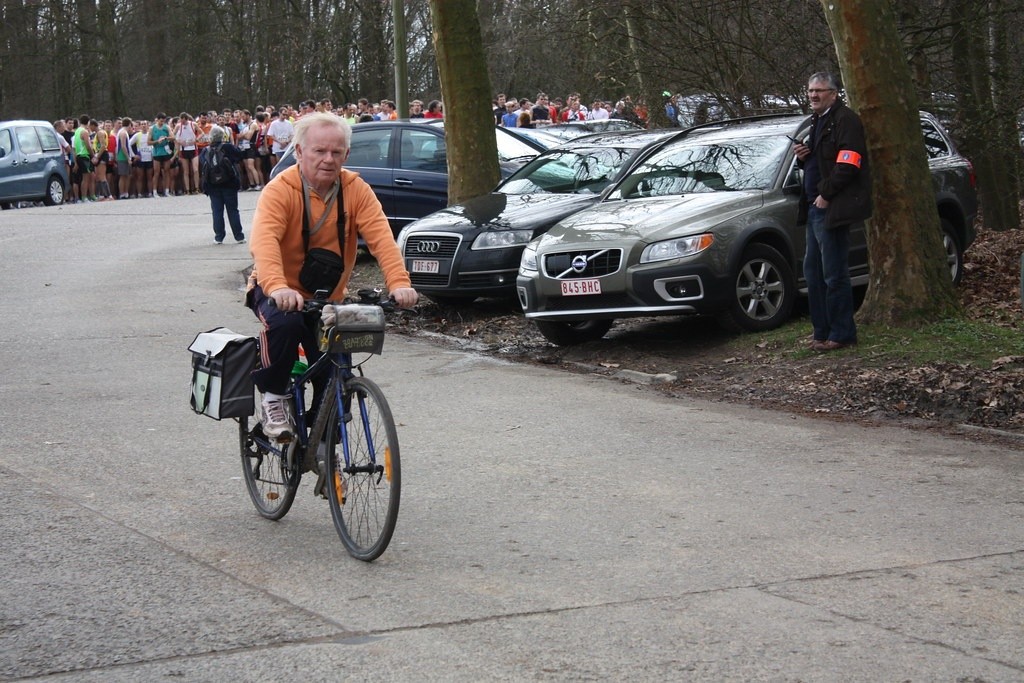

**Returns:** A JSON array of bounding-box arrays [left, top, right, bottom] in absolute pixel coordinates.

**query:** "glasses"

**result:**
[[807, 88, 836, 93]]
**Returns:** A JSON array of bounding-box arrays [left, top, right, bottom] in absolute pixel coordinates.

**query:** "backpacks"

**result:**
[[203, 142, 238, 187]]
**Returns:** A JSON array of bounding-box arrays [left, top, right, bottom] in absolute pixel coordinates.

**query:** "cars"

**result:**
[[396, 126, 694, 314], [273, 115, 651, 262], [1, 120, 70, 206], [517, 107, 983, 347]]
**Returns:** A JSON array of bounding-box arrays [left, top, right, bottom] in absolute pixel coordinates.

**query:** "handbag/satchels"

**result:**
[[299, 171, 345, 298], [188, 326, 258, 421], [316, 304, 384, 356]]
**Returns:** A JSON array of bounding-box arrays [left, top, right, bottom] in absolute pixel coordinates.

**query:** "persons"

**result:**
[[789, 70, 877, 355], [50, 89, 679, 205], [242, 109, 421, 502], [199, 126, 248, 246]]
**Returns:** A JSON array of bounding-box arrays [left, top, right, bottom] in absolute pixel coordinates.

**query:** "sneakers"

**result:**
[[312, 454, 348, 497], [261, 393, 295, 441], [62, 185, 262, 206], [213, 240, 222, 245], [236, 239, 247, 244]]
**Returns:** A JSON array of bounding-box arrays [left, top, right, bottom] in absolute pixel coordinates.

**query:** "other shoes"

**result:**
[[814, 340, 847, 351], [807, 338, 827, 349]]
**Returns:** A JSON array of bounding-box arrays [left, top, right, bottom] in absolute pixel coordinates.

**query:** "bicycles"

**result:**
[[236, 286, 423, 562]]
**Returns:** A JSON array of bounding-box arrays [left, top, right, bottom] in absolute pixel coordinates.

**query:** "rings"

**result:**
[[282, 299, 290, 303]]
